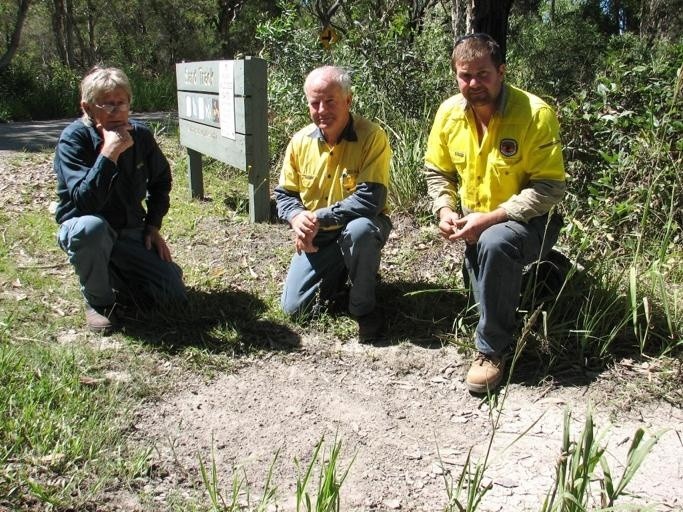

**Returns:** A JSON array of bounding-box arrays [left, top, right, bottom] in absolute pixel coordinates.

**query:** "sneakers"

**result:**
[[348, 293, 375, 316]]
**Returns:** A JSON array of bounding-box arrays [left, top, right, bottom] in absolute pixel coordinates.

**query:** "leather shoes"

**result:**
[[467, 351, 505, 394], [542, 249, 577, 281], [83, 299, 114, 337]]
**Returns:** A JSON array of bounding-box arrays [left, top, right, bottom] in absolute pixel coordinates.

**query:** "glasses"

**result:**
[[94, 103, 129, 112]]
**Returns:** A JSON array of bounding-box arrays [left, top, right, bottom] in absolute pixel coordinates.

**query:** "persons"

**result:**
[[425, 34, 573, 395], [273, 66, 392, 323], [54, 67, 188, 336]]
